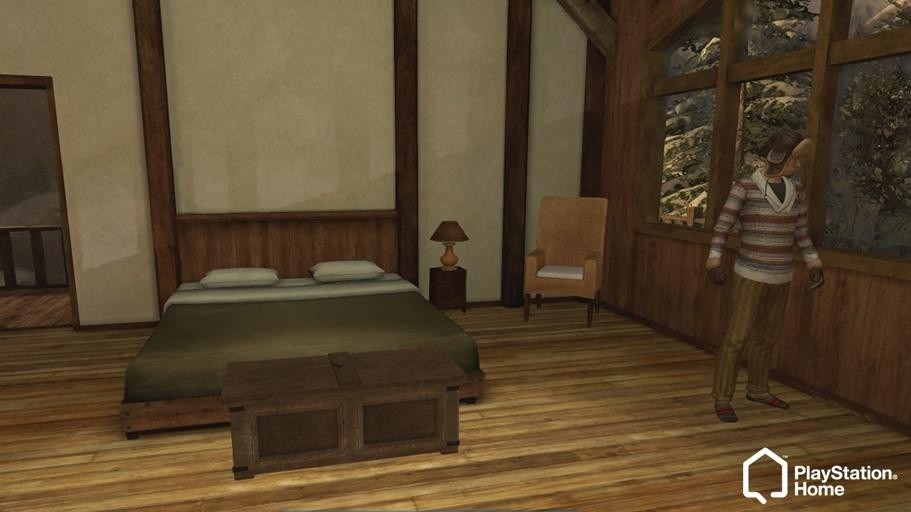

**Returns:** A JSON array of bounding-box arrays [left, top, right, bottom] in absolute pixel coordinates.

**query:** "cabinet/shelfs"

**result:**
[[220, 346, 468, 481]]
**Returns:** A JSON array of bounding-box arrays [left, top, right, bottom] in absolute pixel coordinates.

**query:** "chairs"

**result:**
[[523, 195, 611, 328]]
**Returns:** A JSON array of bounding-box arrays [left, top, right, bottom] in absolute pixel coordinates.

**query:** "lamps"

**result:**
[[431, 220, 471, 272]]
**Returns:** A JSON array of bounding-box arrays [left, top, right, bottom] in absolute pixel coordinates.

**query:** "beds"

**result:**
[[118, 211, 487, 441]]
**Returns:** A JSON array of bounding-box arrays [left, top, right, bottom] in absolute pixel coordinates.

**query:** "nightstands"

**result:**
[[429, 267, 468, 312]]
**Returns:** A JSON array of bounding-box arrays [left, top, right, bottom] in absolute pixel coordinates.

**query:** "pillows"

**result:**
[[199, 264, 281, 289], [307, 258, 388, 283]]
